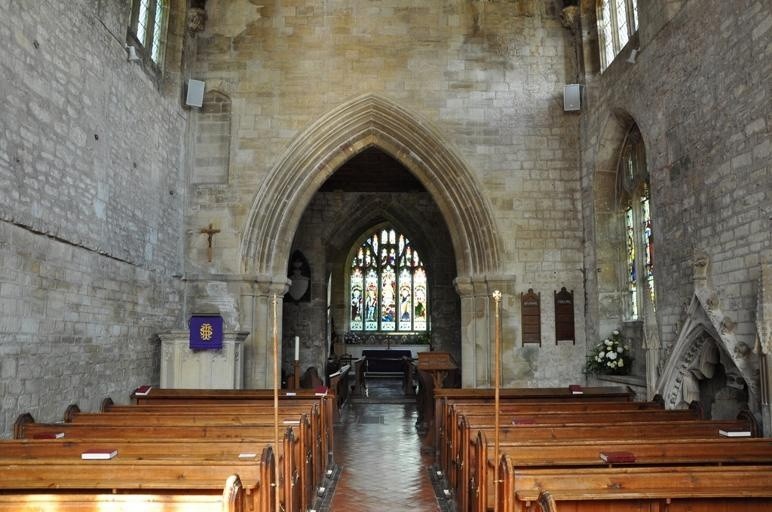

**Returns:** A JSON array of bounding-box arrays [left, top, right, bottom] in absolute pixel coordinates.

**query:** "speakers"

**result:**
[[186, 78, 205, 107], [563, 84, 581, 111]]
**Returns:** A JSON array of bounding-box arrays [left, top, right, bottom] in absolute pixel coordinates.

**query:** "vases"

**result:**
[[618, 358, 634, 375]]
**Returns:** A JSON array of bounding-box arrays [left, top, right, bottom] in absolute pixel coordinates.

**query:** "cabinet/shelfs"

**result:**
[[157, 330, 249, 390]]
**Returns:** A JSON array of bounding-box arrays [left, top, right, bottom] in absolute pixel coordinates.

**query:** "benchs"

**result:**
[[361, 350, 412, 398], [347, 356, 366, 395], [329, 365, 350, 421], [402, 355, 416, 395]]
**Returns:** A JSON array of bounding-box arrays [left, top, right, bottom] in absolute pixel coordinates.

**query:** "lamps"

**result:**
[[124, 42, 139, 61], [625, 47, 641, 64], [289, 261, 310, 301]]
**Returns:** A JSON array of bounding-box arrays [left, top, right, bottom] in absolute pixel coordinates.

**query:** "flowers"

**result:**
[[582, 329, 625, 373]]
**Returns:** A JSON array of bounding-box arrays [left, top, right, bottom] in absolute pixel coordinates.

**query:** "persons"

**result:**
[[364, 282, 379, 323], [381, 280, 395, 323], [350, 285, 364, 324], [412, 283, 426, 323], [398, 283, 410, 322]]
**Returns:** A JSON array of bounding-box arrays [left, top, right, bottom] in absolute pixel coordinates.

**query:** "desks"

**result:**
[[410, 362, 458, 435]]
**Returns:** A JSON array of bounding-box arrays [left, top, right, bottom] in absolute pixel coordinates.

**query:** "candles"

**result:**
[[295, 336, 300, 360]]
